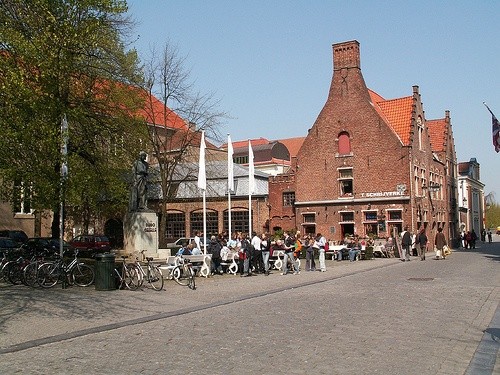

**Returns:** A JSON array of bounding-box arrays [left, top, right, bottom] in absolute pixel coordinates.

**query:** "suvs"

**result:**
[[0, 230, 29, 271]]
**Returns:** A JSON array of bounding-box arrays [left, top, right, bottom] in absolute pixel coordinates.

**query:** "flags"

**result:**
[[226, 136, 235, 193], [248, 142, 255, 195], [491, 114, 500, 153], [198, 131, 208, 191]]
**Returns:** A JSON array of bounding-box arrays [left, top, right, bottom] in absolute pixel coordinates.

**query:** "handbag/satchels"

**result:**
[[325, 242, 329, 251]]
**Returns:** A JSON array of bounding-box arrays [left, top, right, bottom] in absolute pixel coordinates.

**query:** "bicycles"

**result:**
[[0, 244, 95, 288], [104, 249, 164, 291], [173, 253, 195, 290]]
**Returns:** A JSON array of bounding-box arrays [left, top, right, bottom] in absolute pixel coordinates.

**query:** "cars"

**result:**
[[68, 234, 113, 258], [174, 238, 211, 250], [25, 238, 75, 275]]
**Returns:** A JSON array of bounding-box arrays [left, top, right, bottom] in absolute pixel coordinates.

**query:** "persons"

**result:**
[[481, 229, 486, 243], [130, 151, 150, 212], [170, 230, 203, 277], [367, 238, 377, 258], [208, 230, 252, 277], [260, 233, 271, 276], [251, 231, 263, 274], [272, 230, 327, 276], [470, 229, 476, 249], [466, 231, 472, 249], [435, 227, 448, 260], [334, 235, 365, 262], [418, 229, 428, 260], [487, 229, 493, 243], [462, 231, 467, 248], [400, 227, 411, 262], [410, 230, 420, 256]]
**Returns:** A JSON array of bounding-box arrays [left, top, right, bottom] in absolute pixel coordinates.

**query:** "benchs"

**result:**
[[325, 245, 386, 260], [166, 250, 287, 278]]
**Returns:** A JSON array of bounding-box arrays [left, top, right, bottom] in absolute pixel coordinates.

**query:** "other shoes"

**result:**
[[437, 256, 439, 260], [265, 270, 269, 275], [252, 272, 257, 275], [247, 273, 252, 276], [242, 273, 248, 277], [280, 273, 286, 275], [293, 272, 300, 275], [442, 257, 445, 260]]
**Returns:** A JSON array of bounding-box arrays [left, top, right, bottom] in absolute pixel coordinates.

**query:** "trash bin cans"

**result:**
[[95, 254, 116, 291]]
